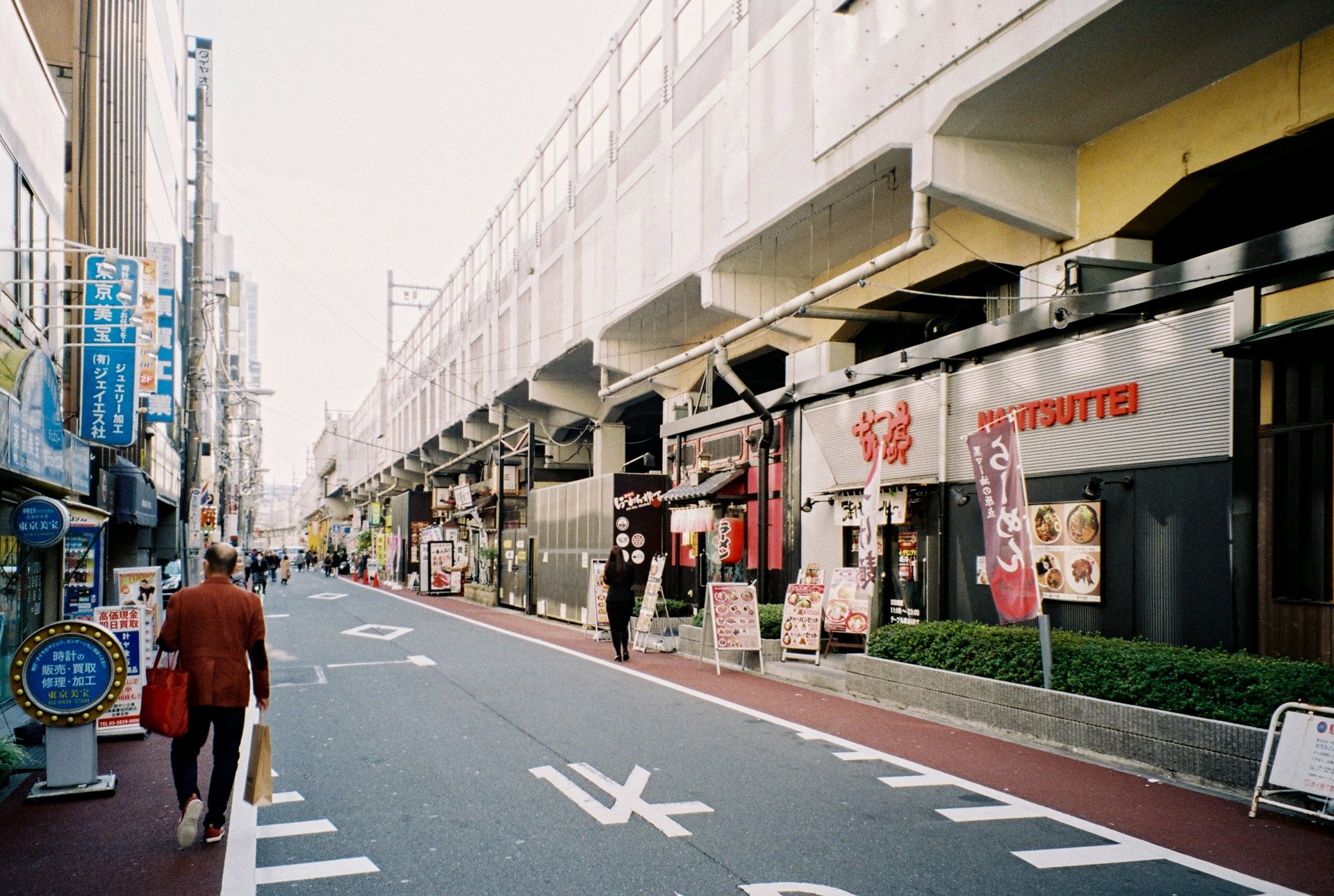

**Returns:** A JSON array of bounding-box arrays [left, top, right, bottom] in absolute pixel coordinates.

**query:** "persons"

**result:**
[[244, 548, 291, 595], [295, 548, 371, 578], [155, 542, 270, 848], [137, 579, 155, 606], [601, 546, 638, 661]]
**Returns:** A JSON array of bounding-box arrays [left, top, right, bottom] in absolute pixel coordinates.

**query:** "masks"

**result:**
[[257, 556, 262, 560]]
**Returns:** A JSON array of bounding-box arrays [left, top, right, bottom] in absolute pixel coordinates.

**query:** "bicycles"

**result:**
[[250, 571, 268, 595]]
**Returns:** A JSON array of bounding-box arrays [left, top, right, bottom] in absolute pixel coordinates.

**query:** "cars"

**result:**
[[284, 546, 306, 565], [158, 558, 182, 605]]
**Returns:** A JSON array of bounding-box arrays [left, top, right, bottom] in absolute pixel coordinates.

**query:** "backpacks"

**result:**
[[325, 554, 331, 564]]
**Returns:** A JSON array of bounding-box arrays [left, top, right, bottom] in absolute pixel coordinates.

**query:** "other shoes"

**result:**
[[325, 572, 336, 578], [271, 579, 288, 586], [623, 652, 629, 661], [615, 654, 621, 662]]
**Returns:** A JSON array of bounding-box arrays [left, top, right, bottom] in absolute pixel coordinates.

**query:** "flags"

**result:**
[[858, 441, 883, 599], [965, 419, 1041, 625]]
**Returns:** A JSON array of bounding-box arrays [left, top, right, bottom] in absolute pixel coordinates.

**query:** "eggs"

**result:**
[[1054, 521, 1060, 532]]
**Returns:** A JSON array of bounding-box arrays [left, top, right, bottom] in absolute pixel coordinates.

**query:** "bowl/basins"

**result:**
[[596, 572, 610, 622], [1067, 504, 1099, 544], [1045, 568, 1063, 591], [782, 591, 822, 648], [1036, 513, 1062, 543], [826, 600, 868, 632]]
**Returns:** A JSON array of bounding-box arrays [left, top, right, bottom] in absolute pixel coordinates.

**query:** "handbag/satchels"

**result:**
[[140, 645, 190, 736], [241, 708, 275, 808]]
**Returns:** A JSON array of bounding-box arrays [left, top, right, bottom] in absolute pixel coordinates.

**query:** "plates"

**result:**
[[714, 591, 756, 647], [1034, 553, 1060, 588], [1064, 554, 1100, 595]]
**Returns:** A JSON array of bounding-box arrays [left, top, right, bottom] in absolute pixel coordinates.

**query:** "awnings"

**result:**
[[657, 468, 745, 502], [454, 494, 497, 518]]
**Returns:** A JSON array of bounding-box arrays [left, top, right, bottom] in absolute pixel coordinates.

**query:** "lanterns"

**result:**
[[717, 517, 745, 565]]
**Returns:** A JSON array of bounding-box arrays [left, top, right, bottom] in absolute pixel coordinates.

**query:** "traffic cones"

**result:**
[[353, 568, 359, 583], [362, 569, 370, 585], [372, 571, 381, 587]]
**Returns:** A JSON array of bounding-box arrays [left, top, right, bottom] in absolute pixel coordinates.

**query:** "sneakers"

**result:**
[[204, 826, 225, 842], [176, 796, 204, 848]]
